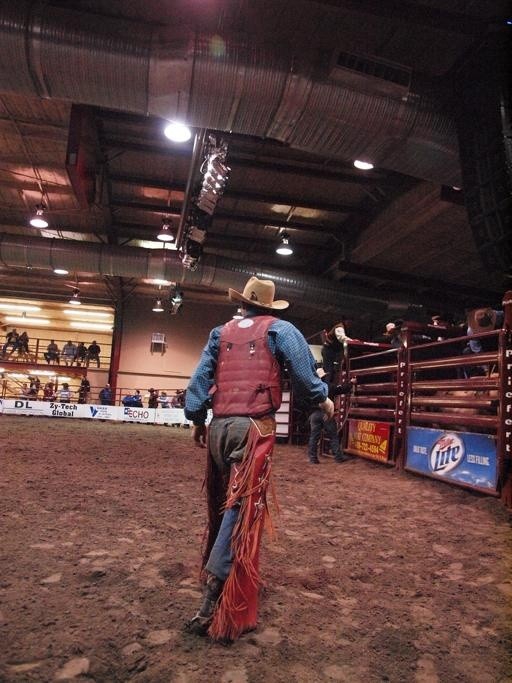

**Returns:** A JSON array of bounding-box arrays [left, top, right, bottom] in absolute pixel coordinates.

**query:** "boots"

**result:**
[[196, 605, 255, 635]]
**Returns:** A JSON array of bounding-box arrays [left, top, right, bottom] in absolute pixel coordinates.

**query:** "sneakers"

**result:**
[[336, 456, 350, 463], [312, 461, 320, 464]]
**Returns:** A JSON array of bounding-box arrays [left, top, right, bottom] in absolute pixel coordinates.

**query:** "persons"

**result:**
[[99, 384, 113, 405], [308, 368, 357, 464], [321, 319, 353, 371], [3, 329, 29, 354], [383, 323, 401, 348], [19, 375, 90, 404], [122, 388, 189, 429], [462, 307, 504, 379], [184, 276, 335, 647], [44, 340, 100, 368]]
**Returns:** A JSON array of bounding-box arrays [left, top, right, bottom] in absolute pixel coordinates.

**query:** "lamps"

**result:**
[[69, 275, 81, 305], [157, 189, 175, 241], [29, 183, 48, 228], [275, 226, 294, 255], [152, 296, 164, 312], [179, 152, 232, 273]]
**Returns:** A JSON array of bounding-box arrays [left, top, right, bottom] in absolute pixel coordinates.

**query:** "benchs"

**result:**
[[0, 343, 111, 365]]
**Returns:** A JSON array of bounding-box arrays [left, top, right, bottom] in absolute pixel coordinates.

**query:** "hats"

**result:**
[[229, 276, 289, 310], [148, 388, 157, 391], [176, 389, 183, 393], [316, 368, 329, 379], [383, 319, 404, 336], [91, 341, 98, 344], [432, 316, 441, 321], [469, 307, 497, 334]]
